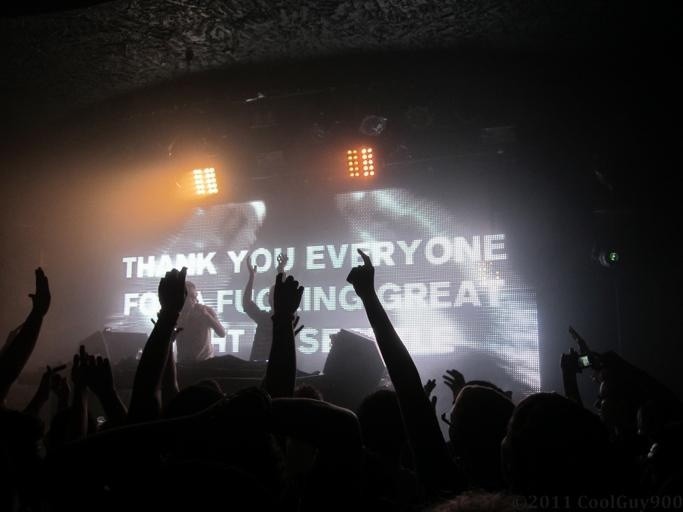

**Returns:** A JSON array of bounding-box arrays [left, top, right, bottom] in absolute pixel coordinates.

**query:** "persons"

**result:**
[[2, 250, 682, 512]]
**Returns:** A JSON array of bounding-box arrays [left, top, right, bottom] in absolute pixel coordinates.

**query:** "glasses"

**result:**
[[441, 412, 452, 425]]
